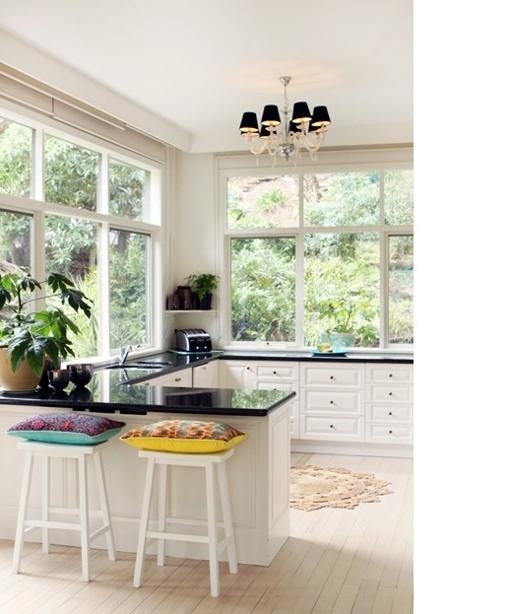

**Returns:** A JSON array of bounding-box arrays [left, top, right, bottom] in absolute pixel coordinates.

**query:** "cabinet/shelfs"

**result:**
[[365, 363, 413, 446], [133, 374, 168, 387], [300, 363, 365, 443], [218, 360, 252, 391], [168, 368, 192, 388], [252, 361, 299, 439], [192, 360, 218, 388]]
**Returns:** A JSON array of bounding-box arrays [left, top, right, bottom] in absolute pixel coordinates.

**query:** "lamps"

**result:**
[[238, 76, 331, 160]]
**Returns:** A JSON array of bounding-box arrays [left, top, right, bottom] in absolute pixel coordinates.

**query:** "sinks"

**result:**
[[108, 361, 175, 370]]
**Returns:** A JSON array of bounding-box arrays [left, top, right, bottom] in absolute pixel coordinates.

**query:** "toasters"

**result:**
[[175, 354, 211, 367], [174, 325, 213, 352]]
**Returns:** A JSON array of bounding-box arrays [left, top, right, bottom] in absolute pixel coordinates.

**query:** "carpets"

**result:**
[[288, 465, 394, 511]]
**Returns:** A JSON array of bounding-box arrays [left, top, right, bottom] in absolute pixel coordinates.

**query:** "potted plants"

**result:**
[[0, 271, 94, 394], [192, 273, 221, 309], [177, 274, 199, 309], [306, 292, 380, 348]]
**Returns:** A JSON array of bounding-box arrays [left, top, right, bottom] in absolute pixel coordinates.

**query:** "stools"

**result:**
[[11, 440, 116, 581], [133, 449, 238, 598]]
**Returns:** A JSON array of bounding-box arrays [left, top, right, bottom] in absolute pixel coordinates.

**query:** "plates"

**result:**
[[309, 347, 349, 354]]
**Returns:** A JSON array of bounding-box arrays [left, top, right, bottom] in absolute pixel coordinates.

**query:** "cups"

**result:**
[[316, 337, 333, 353], [47, 369, 71, 391], [66, 364, 95, 387]]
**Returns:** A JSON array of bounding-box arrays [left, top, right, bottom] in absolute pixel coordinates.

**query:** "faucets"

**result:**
[[119, 370, 133, 390], [118, 347, 133, 365]]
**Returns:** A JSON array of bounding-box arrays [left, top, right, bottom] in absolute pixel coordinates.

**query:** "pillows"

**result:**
[[118, 420, 247, 452], [5, 411, 127, 444]]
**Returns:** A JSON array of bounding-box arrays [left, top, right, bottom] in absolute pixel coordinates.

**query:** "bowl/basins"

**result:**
[[67, 387, 93, 402], [48, 391, 68, 402]]
